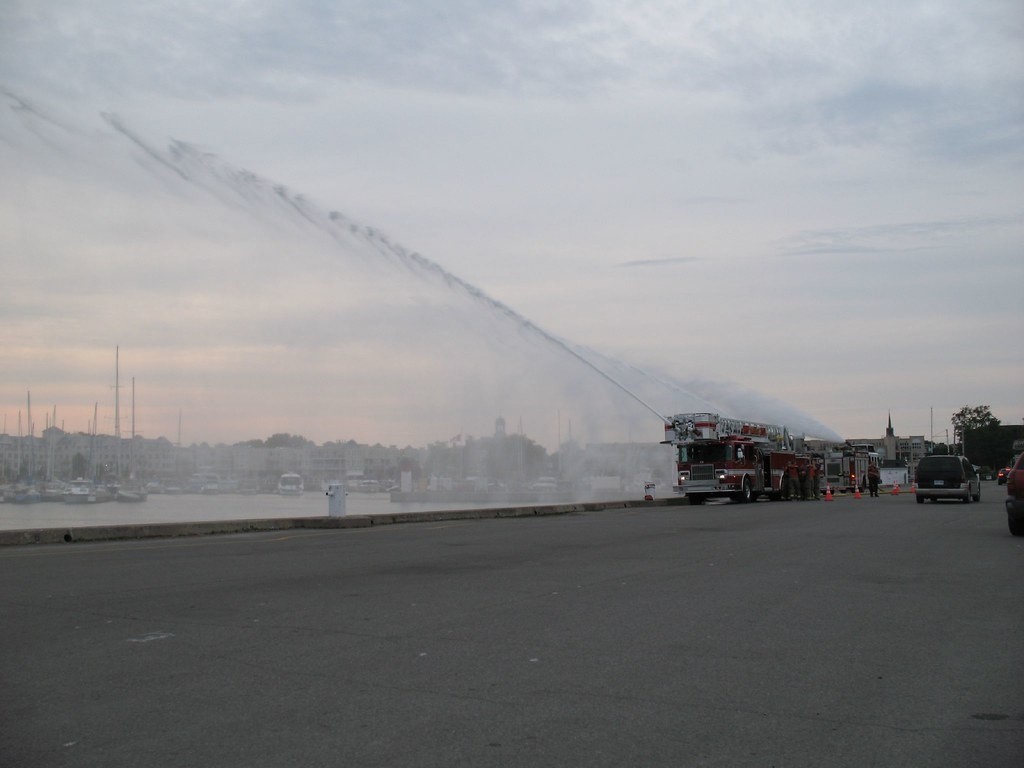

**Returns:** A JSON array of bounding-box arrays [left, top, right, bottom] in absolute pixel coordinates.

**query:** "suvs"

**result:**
[[915, 455, 981, 504]]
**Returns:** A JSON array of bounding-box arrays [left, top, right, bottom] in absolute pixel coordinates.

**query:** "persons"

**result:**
[[785, 460, 823, 501], [868, 461, 880, 497]]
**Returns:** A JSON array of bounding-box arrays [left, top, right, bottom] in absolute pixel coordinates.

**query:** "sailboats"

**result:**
[[0, 343, 305, 506]]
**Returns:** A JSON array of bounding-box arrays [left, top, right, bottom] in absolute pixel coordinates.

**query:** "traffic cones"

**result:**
[[891, 480, 901, 496], [824, 481, 834, 502], [853, 483, 862, 499], [910, 480, 917, 493]]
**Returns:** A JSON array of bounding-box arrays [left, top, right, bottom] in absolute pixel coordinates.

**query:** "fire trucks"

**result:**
[[660, 410, 884, 505]]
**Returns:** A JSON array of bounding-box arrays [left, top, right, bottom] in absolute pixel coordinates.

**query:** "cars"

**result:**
[[1004, 451, 1024, 536], [976, 465, 998, 481], [998, 467, 1013, 485]]
